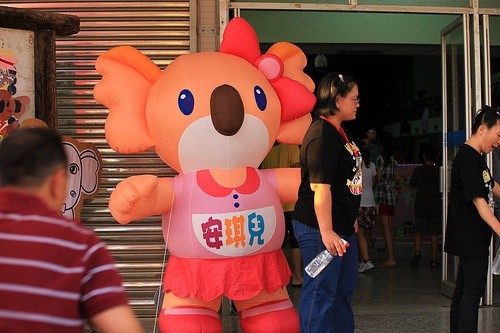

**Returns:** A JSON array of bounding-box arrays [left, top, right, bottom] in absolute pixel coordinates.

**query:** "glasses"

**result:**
[[338, 94, 359, 103]]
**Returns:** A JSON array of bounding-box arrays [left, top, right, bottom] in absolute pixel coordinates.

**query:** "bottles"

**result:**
[[304, 238, 350, 278], [491, 246, 500, 275]]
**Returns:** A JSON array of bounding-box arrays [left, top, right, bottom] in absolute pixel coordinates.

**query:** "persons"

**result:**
[[448, 105, 500, 333], [409, 145, 442, 268], [292, 72, 363, 333], [260, 129, 304, 287], [374, 136, 400, 269], [357, 141, 377, 273], [0, 124, 145, 333]]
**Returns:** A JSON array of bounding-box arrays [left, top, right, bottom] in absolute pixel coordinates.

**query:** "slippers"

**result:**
[[291, 282, 303, 288]]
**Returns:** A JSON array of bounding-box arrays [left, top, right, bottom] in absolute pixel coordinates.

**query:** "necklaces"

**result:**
[[319, 113, 362, 177]]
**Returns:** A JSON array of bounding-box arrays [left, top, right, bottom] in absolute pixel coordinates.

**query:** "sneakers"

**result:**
[[358, 259, 374, 272]]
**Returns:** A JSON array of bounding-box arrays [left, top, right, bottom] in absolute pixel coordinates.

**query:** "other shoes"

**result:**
[[430, 261, 438, 268], [384, 259, 397, 268], [414, 251, 421, 256]]
